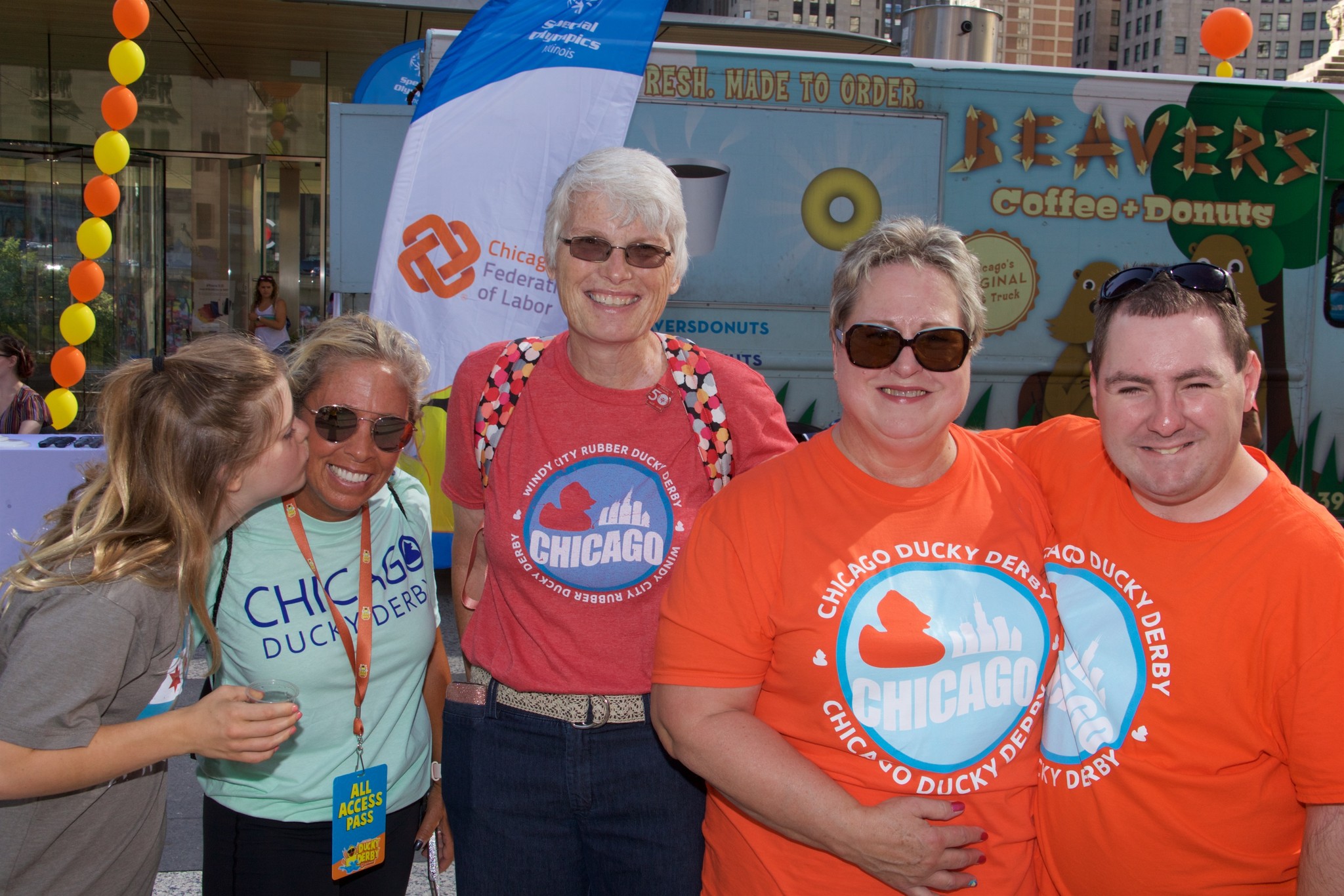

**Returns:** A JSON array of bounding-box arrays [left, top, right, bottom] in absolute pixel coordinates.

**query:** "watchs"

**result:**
[[256, 316, 261, 321]]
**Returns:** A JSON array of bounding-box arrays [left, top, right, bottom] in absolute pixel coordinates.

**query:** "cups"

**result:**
[[245, 679, 300, 705]]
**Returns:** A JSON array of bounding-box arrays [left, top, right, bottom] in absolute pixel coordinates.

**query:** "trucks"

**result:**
[[327, 27, 1344, 589]]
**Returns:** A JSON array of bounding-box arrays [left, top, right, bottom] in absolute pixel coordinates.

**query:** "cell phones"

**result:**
[[426, 830, 440, 896]]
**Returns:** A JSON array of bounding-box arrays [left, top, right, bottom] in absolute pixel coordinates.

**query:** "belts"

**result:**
[[469, 667, 647, 727]]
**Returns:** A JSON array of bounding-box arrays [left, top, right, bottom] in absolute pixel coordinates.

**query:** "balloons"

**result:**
[[44, 0, 150, 430], [1200, 7, 1253, 61], [1216, 61, 1233, 78]]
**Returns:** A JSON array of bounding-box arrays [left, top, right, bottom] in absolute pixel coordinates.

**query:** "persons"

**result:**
[[182, 312, 454, 896], [440, 148, 800, 896], [652, 211, 1060, 896], [248, 275, 291, 360], [0, 332, 53, 434], [965, 262, 1344, 896], [0, 332, 309, 896]]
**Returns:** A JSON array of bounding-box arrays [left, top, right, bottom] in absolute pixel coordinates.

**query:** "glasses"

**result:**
[[1098, 261, 1241, 308], [558, 234, 674, 270], [833, 323, 975, 374], [259, 276, 273, 280], [303, 403, 414, 453]]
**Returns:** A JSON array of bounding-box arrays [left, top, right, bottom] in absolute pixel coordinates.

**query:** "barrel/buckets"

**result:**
[[899, 4, 1004, 63]]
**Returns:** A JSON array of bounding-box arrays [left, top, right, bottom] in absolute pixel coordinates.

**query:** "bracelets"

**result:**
[[430, 761, 442, 781]]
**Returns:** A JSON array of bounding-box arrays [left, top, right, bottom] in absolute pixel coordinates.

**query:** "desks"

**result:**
[[0, 432, 110, 615]]
